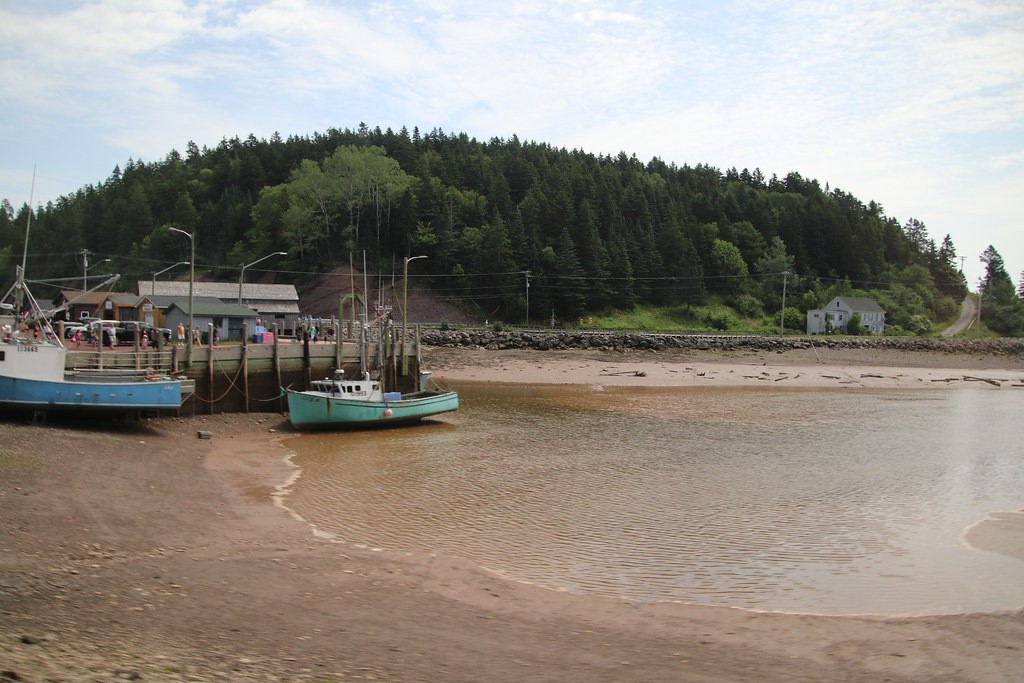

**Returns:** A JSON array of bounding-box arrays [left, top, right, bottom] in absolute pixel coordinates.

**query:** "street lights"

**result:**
[[238, 252, 289, 308], [169, 226, 195, 367], [151, 260, 192, 305], [401, 254, 428, 377]]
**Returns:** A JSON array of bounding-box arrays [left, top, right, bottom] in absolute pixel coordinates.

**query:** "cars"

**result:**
[[70, 319, 121, 341]]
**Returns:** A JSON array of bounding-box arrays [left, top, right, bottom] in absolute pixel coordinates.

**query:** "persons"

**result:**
[[3, 318, 336, 350]]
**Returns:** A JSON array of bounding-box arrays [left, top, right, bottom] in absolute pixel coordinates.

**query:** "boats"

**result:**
[[282, 249, 461, 433], [0, 158, 184, 416]]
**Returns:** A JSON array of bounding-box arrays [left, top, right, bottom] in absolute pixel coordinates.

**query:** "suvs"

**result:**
[[53, 317, 100, 338]]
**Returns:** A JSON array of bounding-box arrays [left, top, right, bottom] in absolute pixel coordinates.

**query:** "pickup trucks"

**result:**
[[102, 319, 173, 348]]
